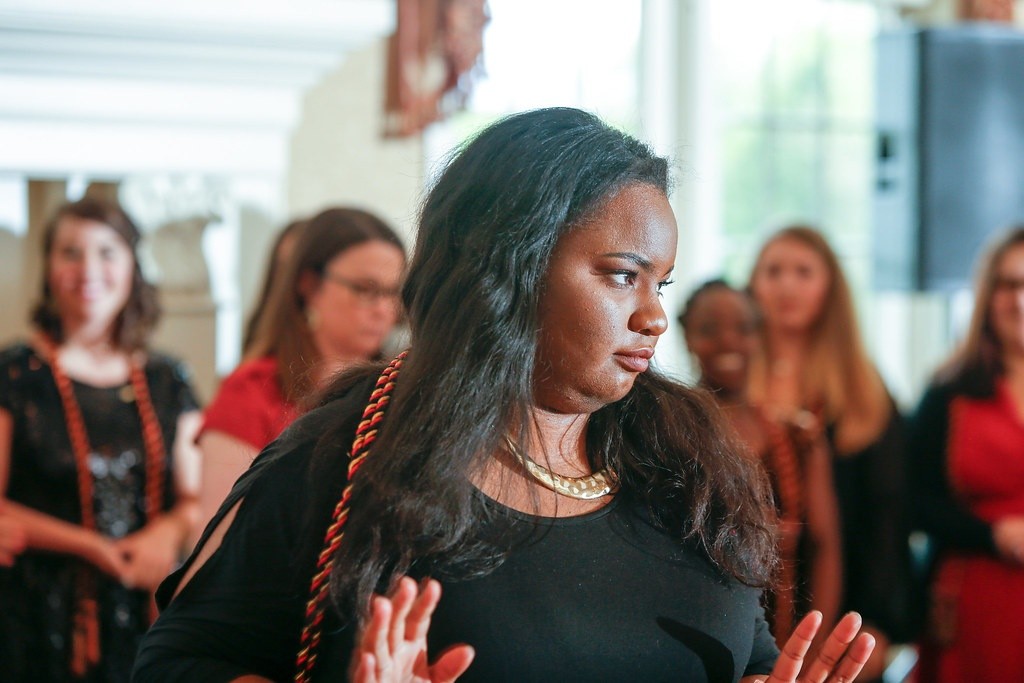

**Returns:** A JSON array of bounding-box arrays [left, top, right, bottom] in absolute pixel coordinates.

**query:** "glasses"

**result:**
[[313, 272, 400, 296], [991, 277, 1024, 291]]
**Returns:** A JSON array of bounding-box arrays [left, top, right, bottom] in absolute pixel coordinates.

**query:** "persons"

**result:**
[[0, 200, 408, 682], [677, 226, 1024, 682], [131, 106, 878, 683]]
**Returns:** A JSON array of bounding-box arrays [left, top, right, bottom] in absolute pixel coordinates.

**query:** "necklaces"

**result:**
[[499, 432, 619, 500]]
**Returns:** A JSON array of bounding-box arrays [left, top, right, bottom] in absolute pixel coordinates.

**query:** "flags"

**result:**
[[387, 0, 492, 137]]
